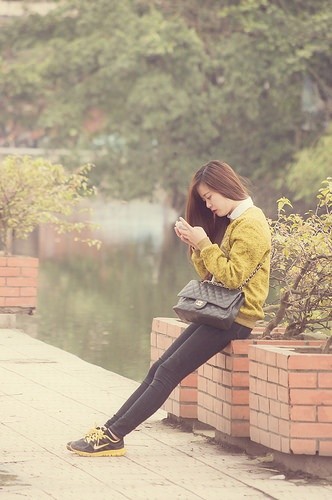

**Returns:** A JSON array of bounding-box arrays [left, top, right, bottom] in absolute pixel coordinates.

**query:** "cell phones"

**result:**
[[175, 221, 188, 230]]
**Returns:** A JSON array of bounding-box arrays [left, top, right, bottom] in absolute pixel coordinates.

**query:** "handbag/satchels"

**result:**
[[172, 279, 246, 330]]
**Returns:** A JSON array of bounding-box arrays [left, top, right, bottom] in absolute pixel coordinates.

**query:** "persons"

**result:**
[[66, 160, 273, 456]]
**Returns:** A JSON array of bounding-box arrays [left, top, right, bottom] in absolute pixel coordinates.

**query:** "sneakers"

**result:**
[[66, 424, 126, 456]]
